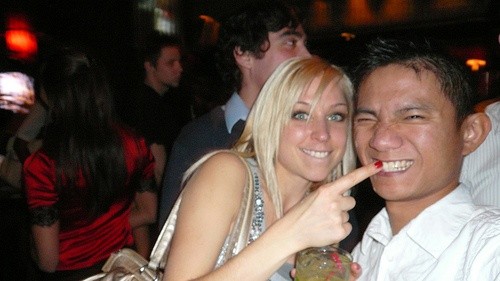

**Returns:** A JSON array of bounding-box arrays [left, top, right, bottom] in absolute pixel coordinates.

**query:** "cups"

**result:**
[[296, 247, 353, 281]]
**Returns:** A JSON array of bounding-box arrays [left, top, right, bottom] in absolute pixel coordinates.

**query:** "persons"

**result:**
[[350, 37, 500, 281], [0, 0, 499, 254], [24, 44, 157, 281], [150, 55, 383, 281]]
